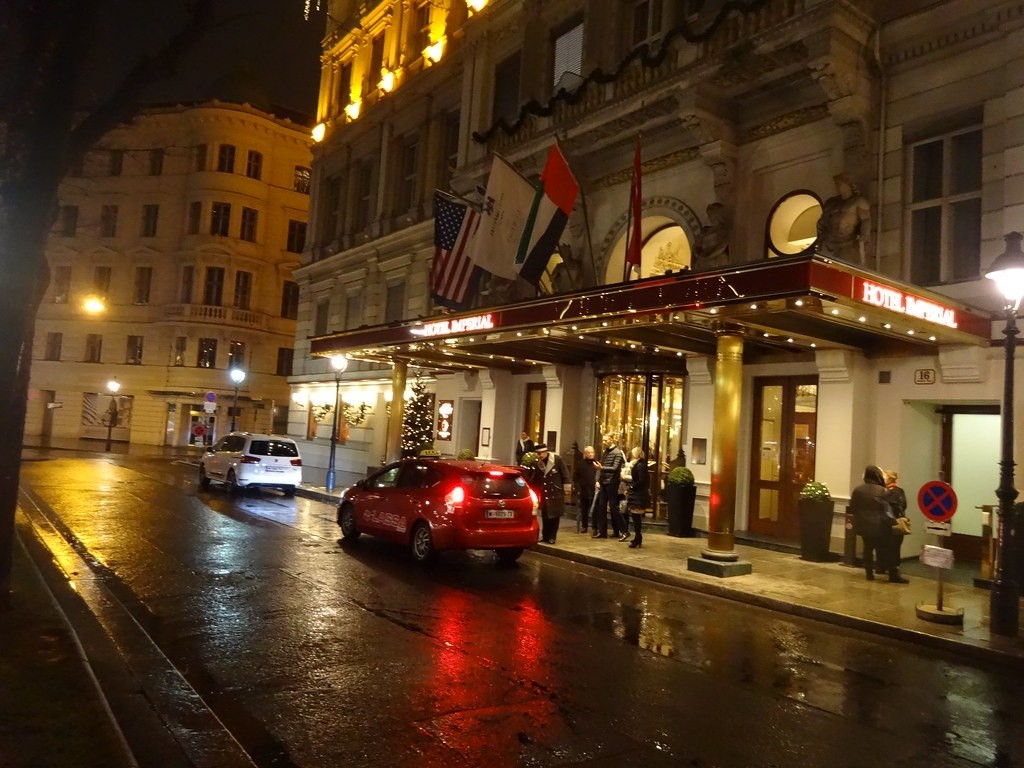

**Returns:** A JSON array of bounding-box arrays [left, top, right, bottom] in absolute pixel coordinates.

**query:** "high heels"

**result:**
[[629, 534, 642, 548]]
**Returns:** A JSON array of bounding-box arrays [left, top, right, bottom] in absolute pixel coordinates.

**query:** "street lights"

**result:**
[[985, 231, 1024, 637], [231, 369, 245, 432], [106, 381, 121, 451], [325, 354, 348, 492]]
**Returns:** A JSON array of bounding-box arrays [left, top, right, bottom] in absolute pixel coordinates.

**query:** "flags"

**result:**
[[431, 196, 482, 310], [628, 133, 642, 267], [473, 157, 536, 280], [514, 142, 579, 288]]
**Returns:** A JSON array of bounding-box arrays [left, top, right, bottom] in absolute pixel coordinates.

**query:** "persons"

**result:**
[[516, 430, 534, 465], [549, 244, 584, 293], [532, 444, 569, 545], [884, 470, 907, 566], [619, 447, 651, 549], [573, 445, 597, 533], [691, 202, 732, 269], [816, 172, 871, 266], [851, 465, 910, 584], [592, 433, 622, 538]]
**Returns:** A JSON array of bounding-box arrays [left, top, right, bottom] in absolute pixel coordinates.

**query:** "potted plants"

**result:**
[[666, 467, 697, 538], [798, 480, 835, 560], [520, 452, 537, 475]]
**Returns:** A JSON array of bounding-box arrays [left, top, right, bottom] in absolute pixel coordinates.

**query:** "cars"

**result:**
[[198, 432, 303, 497], [336, 449, 540, 566]]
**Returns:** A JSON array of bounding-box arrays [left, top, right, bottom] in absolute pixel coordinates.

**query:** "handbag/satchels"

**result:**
[[618, 449, 633, 482], [891, 516, 911, 535]]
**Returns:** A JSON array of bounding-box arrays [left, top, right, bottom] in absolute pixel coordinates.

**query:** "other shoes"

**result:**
[[548, 538, 555, 543], [865, 567, 874, 580], [538, 537, 549, 542], [619, 532, 631, 541], [610, 533, 620, 538], [875, 569, 890, 574], [580, 527, 588, 533], [889, 575, 909, 584], [592, 533, 607, 538]]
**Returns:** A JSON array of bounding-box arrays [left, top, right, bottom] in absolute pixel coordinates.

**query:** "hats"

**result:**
[[534, 444, 547, 452]]
